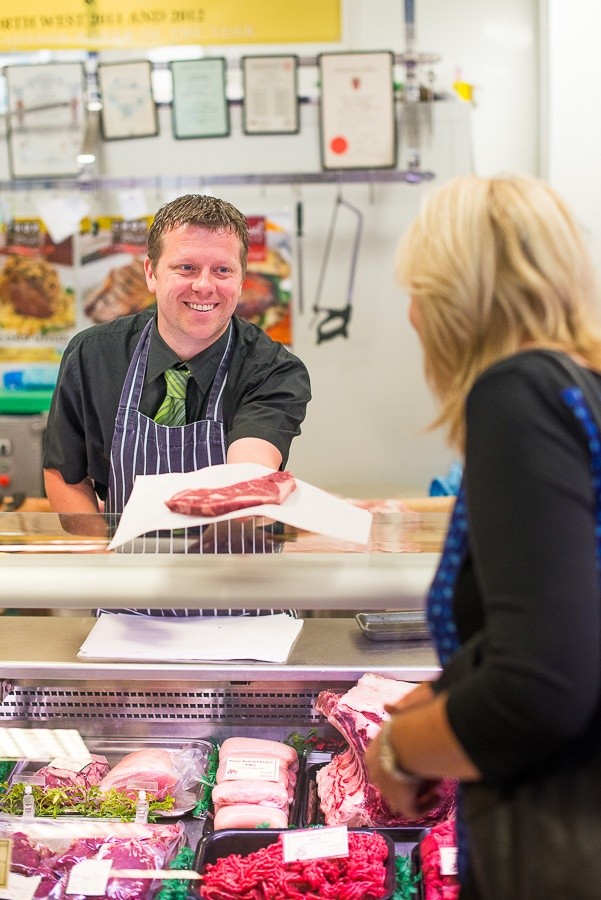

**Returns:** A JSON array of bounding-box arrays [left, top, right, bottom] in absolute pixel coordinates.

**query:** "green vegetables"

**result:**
[[0, 781, 176, 825]]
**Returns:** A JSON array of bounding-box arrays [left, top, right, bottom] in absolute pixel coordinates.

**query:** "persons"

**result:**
[[41, 196, 314, 615], [362, 173, 601, 900]]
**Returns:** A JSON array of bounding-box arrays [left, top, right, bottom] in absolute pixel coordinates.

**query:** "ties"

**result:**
[[152, 367, 195, 427]]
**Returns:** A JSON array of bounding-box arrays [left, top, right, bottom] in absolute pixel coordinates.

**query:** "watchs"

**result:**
[[376, 718, 425, 786]]
